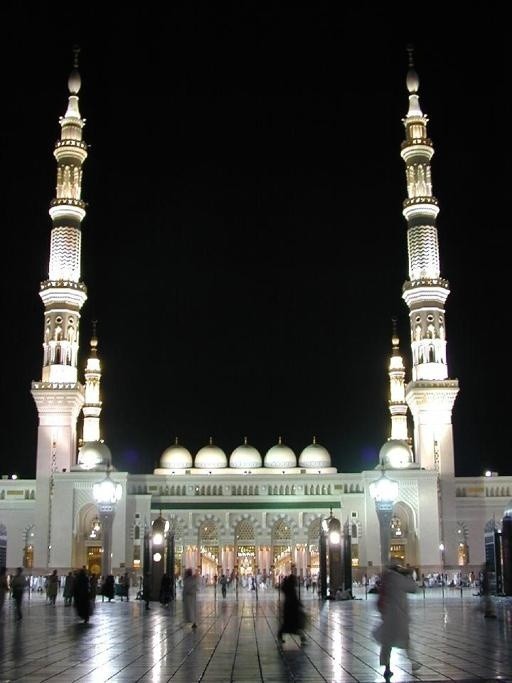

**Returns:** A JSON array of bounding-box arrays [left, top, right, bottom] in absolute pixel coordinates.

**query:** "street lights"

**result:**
[[24, 524, 36, 600]]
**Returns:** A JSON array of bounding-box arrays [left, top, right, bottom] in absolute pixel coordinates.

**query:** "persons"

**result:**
[[275, 563, 310, 646], [1, 562, 176, 627], [180, 567, 199, 629], [218, 573, 227, 597], [373, 560, 423, 679]]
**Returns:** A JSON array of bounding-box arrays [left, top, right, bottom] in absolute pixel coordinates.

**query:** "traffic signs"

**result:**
[[92, 460, 123, 594], [370, 459, 398, 575], [456, 519, 469, 600]]
